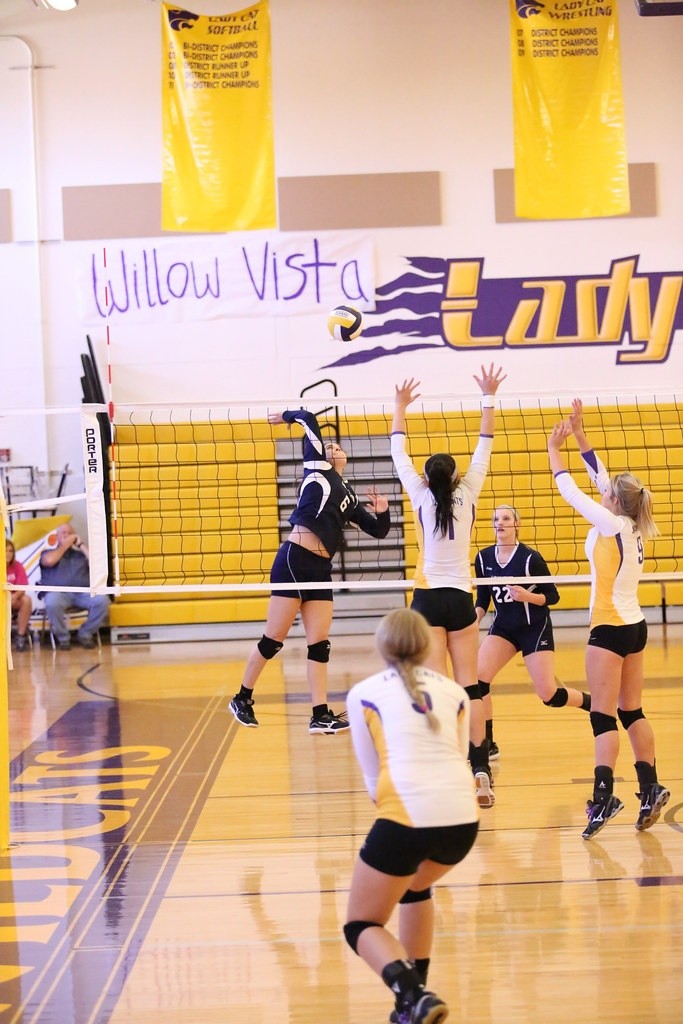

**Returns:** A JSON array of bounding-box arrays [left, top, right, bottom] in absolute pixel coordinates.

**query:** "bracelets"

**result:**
[[77, 542, 84, 548], [483, 394, 494, 408]]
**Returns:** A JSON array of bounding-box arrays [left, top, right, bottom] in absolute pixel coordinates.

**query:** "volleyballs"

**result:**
[[326, 305, 363, 342]]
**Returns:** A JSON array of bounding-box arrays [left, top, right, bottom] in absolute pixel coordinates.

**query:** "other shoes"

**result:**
[[390, 984, 449, 1024], [16, 635, 25, 651], [467, 743, 500, 763], [60, 641, 70, 649], [472, 765, 495, 808], [76, 636, 94, 647]]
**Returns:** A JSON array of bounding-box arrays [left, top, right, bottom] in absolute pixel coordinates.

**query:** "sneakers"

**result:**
[[228, 694, 258, 727], [581, 794, 624, 841], [309, 710, 349, 734], [635, 782, 670, 831]]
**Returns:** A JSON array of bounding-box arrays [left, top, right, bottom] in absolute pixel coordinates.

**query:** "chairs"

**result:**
[[11, 606, 102, 652]]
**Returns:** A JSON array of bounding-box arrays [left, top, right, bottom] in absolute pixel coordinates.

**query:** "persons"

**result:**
[[472, 504, 593, 761], [342, 610, 480, 1024], [230, 409, 392, 735], [548, 397, 673, 839], [39, 525, 109, 650], [5, 538, 32, 651], [389, 364, 508, 810]]
